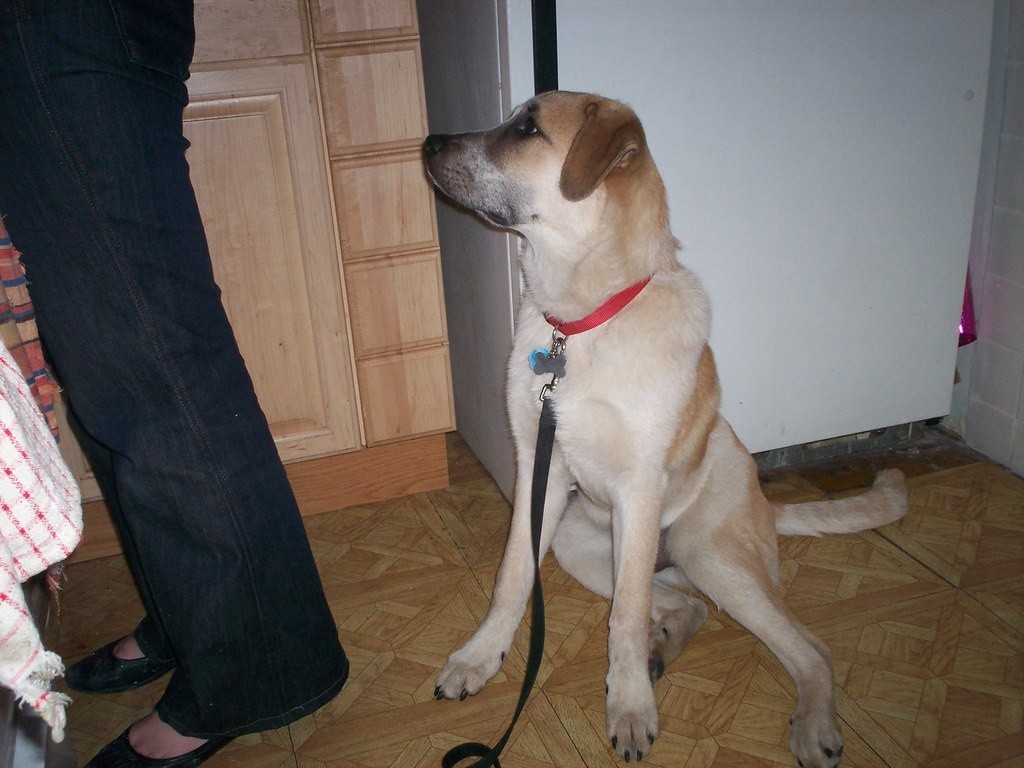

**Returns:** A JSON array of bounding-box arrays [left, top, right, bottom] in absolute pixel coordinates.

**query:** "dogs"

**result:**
[[423, 91, 909, 768]]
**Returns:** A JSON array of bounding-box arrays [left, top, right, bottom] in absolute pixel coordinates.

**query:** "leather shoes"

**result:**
[[64, 634, 177, 695], [83, 715, 237, 768]]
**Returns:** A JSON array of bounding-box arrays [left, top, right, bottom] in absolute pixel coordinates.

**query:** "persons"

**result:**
[[0, 0, 350, 768]]
